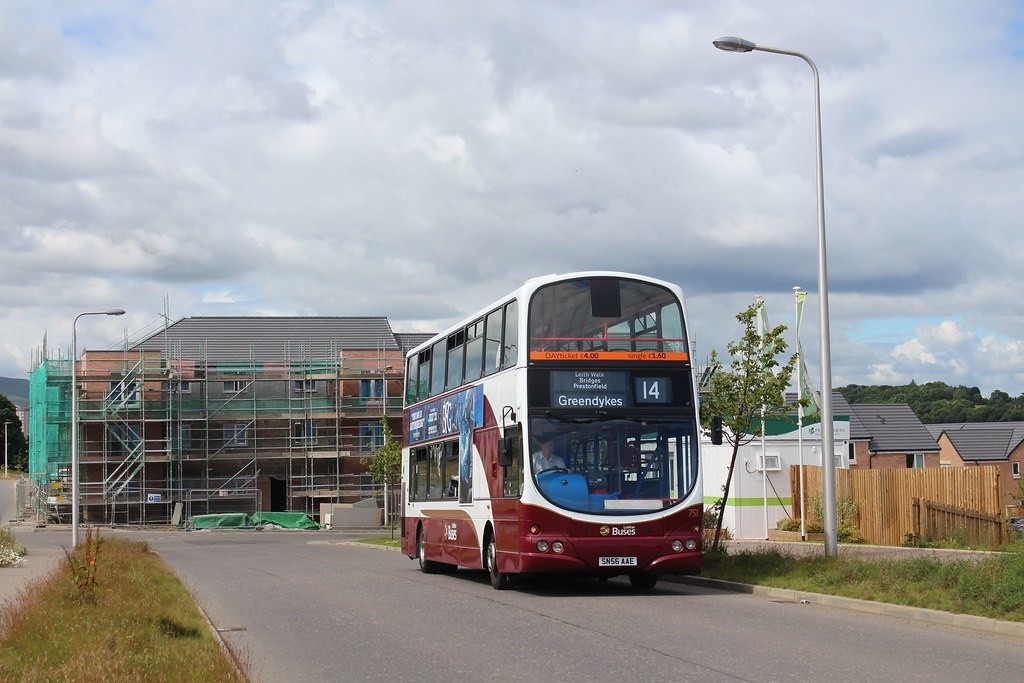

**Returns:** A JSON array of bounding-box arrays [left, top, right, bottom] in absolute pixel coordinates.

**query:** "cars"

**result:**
[[1012, 518, 1024, 534]]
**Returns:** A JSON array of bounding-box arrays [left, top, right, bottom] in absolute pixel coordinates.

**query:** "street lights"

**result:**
[[3, 422, 13, 478], [382, 364, 394, 526], [714, 35, 836, 561], [69, 309, 126, 549]]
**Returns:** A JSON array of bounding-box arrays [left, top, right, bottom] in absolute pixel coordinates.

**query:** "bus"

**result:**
[[399, 270, 724, 595]]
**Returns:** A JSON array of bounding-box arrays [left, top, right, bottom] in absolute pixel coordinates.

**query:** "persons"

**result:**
[[460, 389, 476, 503], [531, 436, 571, 475]]
